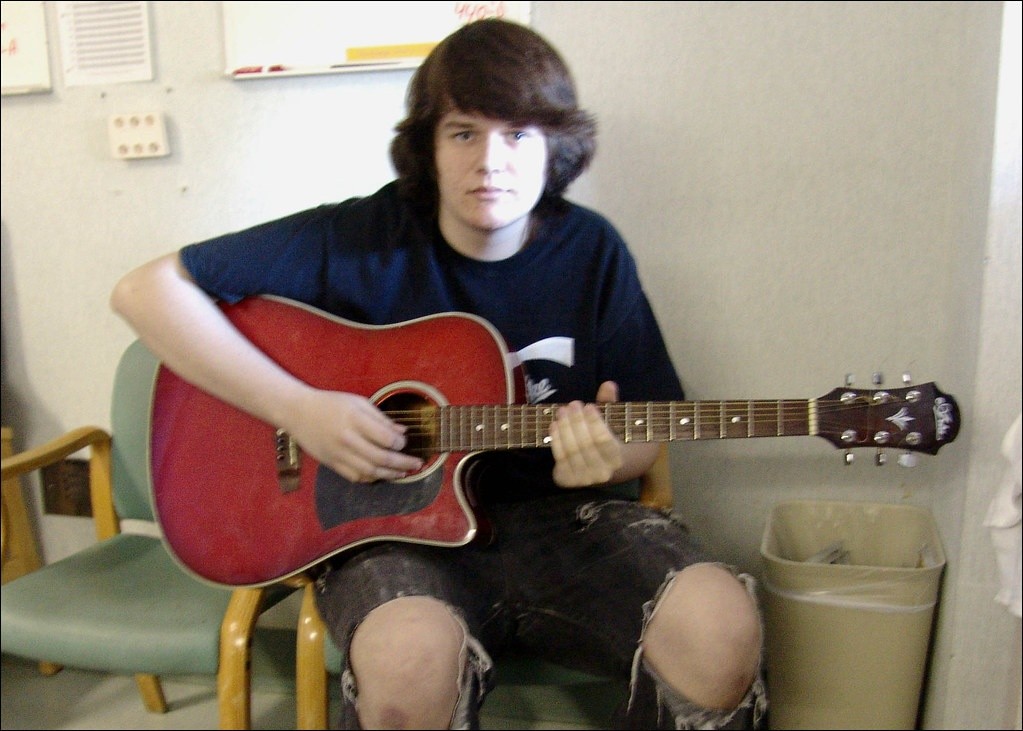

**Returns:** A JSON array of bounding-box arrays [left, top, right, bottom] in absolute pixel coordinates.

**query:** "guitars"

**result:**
[[144, 292, 964, 592]]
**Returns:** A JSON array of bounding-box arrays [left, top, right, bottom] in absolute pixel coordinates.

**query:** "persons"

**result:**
[[109, 19, 765, 731]]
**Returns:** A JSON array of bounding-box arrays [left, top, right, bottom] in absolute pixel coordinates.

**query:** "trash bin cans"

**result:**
[[758, 499, 947, 731]]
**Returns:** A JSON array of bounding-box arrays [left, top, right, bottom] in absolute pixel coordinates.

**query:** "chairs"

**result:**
[[296, 442, 670, 730], [1, 335, 299, 731]]
